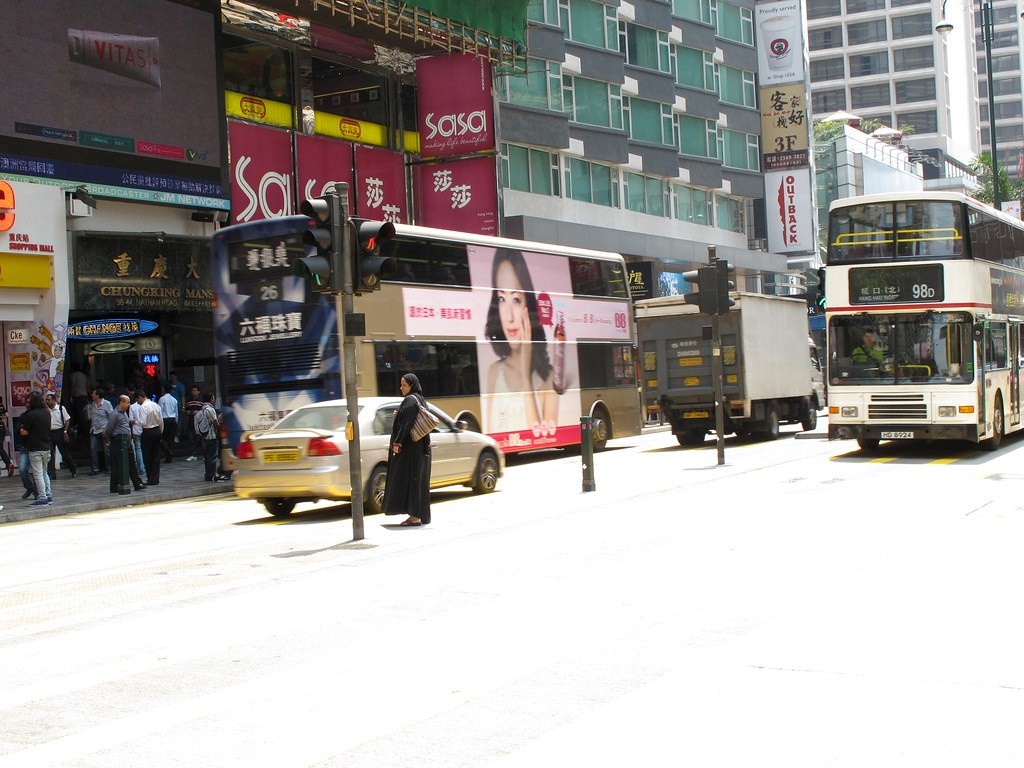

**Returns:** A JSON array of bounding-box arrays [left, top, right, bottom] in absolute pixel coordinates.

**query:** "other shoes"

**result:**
[[22, 487, 33, 499], [29, 498, 49, 507], [119, 486, 132, 494], [45, 495, 53, 505], [143, 480, 159, 485], [134, 484, 147, 490], [110, 487, 120, 493], [73, 468, 80, 478], [8, 464, 14, 477]]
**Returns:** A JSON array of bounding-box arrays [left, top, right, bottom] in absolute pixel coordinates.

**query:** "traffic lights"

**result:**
[[293, 194, 343, 294], [816, 270, 826, 313], [717, 259, 736, 314], [682, 267, 718, 315], [351, 218, 396, 293]]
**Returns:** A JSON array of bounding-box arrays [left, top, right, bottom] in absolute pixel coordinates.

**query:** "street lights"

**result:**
[[935, 0, 1001, 210]]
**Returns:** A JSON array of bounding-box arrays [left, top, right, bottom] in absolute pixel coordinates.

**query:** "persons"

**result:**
[[382, 345, 473, 394], [850, 329, 884, 371], [70, 361, 88, 433], [395, 262, 471, 285], [18, 391, 53, 507], [186, 385, 205, 461], [380, 373, 430, 526], [485, 247, 558, 434], [88, 377, 178, 493], [46, 393, 78, 479], [0, 403, 14, 477], [169, 371, 186, 443], [202, 391, 234, 483]]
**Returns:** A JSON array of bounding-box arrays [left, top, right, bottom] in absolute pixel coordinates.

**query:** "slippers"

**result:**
[[400, 516, 422, 526]]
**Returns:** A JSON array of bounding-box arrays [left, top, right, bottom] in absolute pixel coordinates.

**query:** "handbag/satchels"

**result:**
[[408, 395, 439, 442], [67, 427, 78, 443]]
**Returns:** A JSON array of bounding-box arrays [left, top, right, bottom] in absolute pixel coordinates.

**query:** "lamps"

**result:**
[[72, 185, 98, 210], [191, 208, 215, 223]]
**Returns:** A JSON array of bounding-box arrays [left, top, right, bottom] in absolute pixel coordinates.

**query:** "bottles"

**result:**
[[552, 311, 567, 395]]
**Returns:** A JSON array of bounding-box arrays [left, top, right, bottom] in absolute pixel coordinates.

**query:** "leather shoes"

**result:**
[[89, 470, 99, 475]]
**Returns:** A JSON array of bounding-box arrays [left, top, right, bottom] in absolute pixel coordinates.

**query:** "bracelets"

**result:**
[[183, 407, 185, 408]]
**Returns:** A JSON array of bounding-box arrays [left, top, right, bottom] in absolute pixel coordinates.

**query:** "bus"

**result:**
[[826, 191, 1024, 451], [211, 217, 642, 470]]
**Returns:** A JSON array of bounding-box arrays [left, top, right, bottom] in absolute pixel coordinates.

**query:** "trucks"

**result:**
[[633, 292, 825, 446]]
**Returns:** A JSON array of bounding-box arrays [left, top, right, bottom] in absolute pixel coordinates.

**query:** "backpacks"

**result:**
[[194, 405, 213, 437]]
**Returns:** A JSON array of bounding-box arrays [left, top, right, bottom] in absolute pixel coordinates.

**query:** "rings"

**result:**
[[64, 430, 67, 433]]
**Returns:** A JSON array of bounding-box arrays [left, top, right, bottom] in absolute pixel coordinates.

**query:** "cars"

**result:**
[[231, 397, 505, 514]]
